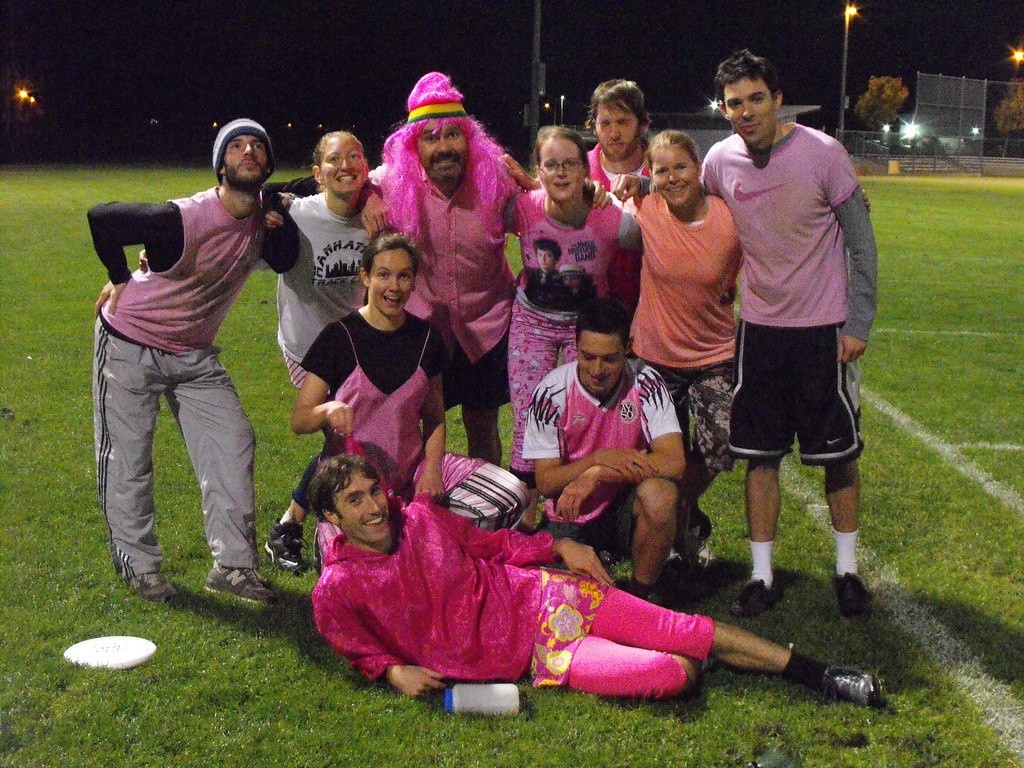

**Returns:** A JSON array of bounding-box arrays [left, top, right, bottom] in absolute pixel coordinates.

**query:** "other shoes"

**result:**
[[688, 499, 712, 540], [630, 588, 664, 604]]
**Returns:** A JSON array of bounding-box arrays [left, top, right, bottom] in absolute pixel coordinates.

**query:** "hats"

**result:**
[[212, 118, 276, 185]]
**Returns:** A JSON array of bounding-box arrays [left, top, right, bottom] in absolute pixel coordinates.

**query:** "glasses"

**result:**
[[538, 157, 583, 176]]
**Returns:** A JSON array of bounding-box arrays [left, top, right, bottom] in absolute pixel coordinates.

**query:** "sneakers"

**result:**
[[204, 561, 277, 605], [264, 518, 311, 578], [125, 572, 178, 604], [728, 577, 783, 617], [821, 666, 879, 707], [314, 527, 321, 575], [831, 566, 872, 618]]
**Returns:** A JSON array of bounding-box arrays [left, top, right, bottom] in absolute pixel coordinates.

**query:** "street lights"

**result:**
[[560, 96, 565, 127], [838, 6, 857, 144], [1002, 50, 1024, 159]]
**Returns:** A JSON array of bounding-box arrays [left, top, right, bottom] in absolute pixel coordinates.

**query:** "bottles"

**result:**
[[443, 684, 519, 719]]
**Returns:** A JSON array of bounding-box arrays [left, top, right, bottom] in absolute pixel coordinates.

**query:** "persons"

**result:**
[[359, 73, 613, 467], [502, 128, 871, 566], [504, 125, 625, 535], [307, 454, 879, 708], [264, 131, 381, 569], [292, 232, 527, 574], [610, 49, 878, 617], [587, 80, 652, 215], [525, 238, 597, 309], [522, 299, 686, 601], [88, 119, 300, 606]]
